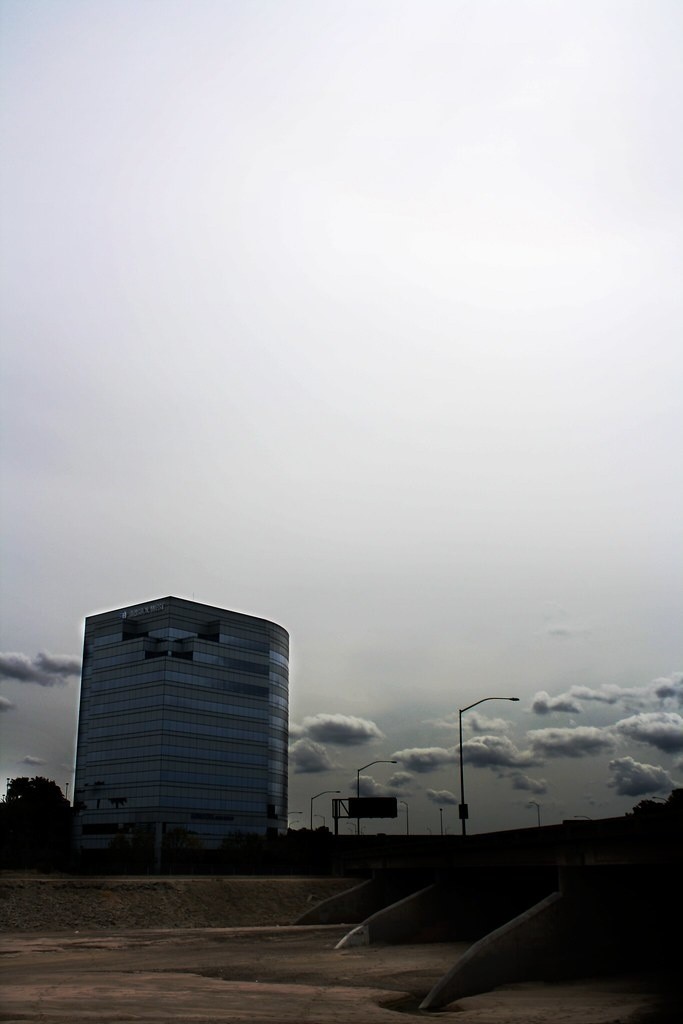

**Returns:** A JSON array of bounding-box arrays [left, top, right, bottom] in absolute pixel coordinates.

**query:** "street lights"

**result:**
[[400, 801, 409, 836], [312, 814, 325, 829], [459, 698, 519, 836], [358, 762, 397, 837], [529, 802, 541, 829], [310, 790, 341, 830], [439, 808, 443, 835]]
